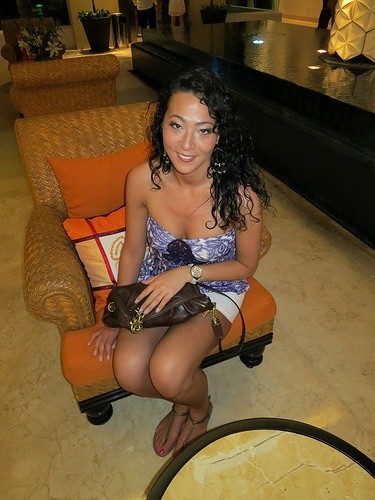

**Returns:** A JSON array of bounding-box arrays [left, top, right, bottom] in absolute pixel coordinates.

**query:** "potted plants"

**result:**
[[200, 2, 229, 24], [78, 8, 111, 52]]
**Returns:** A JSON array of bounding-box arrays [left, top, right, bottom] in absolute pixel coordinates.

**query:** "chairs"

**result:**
[[10, 54, 119, 118], [14, 100, 276, 426], [1, 17, 66, 70]]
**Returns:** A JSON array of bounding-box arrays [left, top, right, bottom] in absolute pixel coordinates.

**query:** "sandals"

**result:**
[[153, 403, 189, 457], [172, 401, 213, 458]]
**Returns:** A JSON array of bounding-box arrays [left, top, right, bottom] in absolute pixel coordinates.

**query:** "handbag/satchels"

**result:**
[[102, 282, 224, 339]]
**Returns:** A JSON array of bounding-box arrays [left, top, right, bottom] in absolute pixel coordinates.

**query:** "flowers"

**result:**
[[13, 18, 65, 62]]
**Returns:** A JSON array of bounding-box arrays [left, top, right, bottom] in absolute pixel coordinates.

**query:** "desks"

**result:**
[[146, 417, 375, 500]]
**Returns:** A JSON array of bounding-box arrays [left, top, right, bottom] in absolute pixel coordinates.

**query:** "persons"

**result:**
[[87, 68, 271, 459], [132, 0, 157, 41]]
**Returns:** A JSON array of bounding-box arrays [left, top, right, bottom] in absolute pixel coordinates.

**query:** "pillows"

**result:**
[[46, 140, 153, 218], [62, 204, 127, 312]]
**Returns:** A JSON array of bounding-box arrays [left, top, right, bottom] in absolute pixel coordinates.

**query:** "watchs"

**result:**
[[188, 264, 202, 284]]
